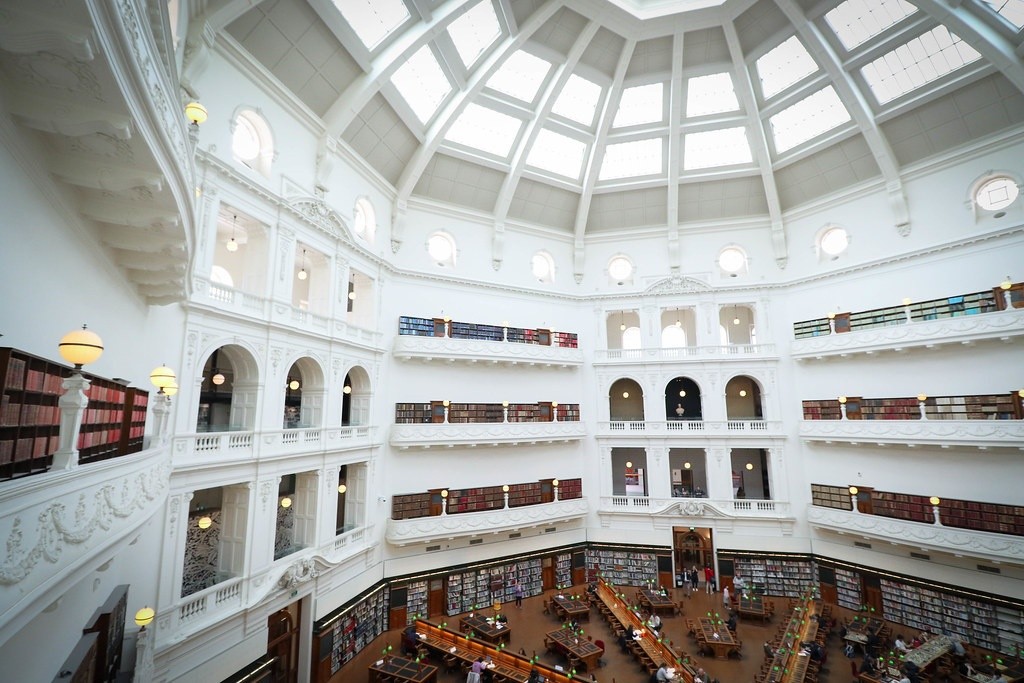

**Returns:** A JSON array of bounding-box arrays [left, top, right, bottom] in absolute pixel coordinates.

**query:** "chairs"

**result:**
[[373, 588, 976, 683]]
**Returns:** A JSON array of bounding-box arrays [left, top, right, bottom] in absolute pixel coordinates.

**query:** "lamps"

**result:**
[[212, 346, 225, 385], [349, 274, 356, 300], [626, 449, 753, 470], [152, 363, 179, 401], [135, 606, 155, 632], [185, 101, 208, 126], [622, 376, 746, 398], [289, 379, 299, 390], [343, 385, 352, 394], [298, 249, 308, 279], [226, 216, 238, 251], [199, 464, 347, 528], [58, 323, 104, 370], [620, 305, 740, 331]]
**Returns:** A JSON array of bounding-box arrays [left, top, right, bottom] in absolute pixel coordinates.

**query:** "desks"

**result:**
[[738, 593, 766, 625], [459, 612, 511, 649], [858, 634, 1024, 683], [549, 593, 590, 624], [369, 654, 439, 683], [698, 617, 736, 661], [639, 587, 676, 618], [841, 615, 887, 658], [545, 628, 603, 674]]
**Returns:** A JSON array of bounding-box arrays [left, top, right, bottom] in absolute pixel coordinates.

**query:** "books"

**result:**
[[331, 587, 389, 674], [0, 357, 148, 465], [585, 550, 656, 586], [558, 553, 571, 588], [791, 292, 1024, 537], [735, 559, 820, 598], [880, 579, 993, 649], [407, 581, 428, 625], [392, 316, 582, 519], [447, 559, 542, 616], [835, 568, 859, 610]]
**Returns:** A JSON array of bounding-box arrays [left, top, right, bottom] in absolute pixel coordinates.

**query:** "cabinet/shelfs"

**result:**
[[443, 549, 659, 617], [0, 346, 149, 484], [392, 478, 582, 520], [52, 585, 128, 683], [733, 556, 1024, 660], [398, 316, 578, 349], [811, 483, 1024, 537], [793, 281, 998, 339], [802, 391, 1016, 420], [331, 580, 428, 678], [395, 402, 580, 424]]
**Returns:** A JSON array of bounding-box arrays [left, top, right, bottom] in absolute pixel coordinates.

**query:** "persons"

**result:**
[[840, 622, 931, 683], [627, 612, 711, 683], [809, 613, 826, 660], [737, 486, 745, 498], [497, 614, 508, 626], [724, 585, 731, 610], [681, 564, 714, 597], [676, 404, 684, 414], [733, 572, 743, 595], [588, 584, 597, 593], [404, 629, 538, 683], [724, 612, 736, 631], [673, 486, 705, 498], [590, 674, 597, 682]]
